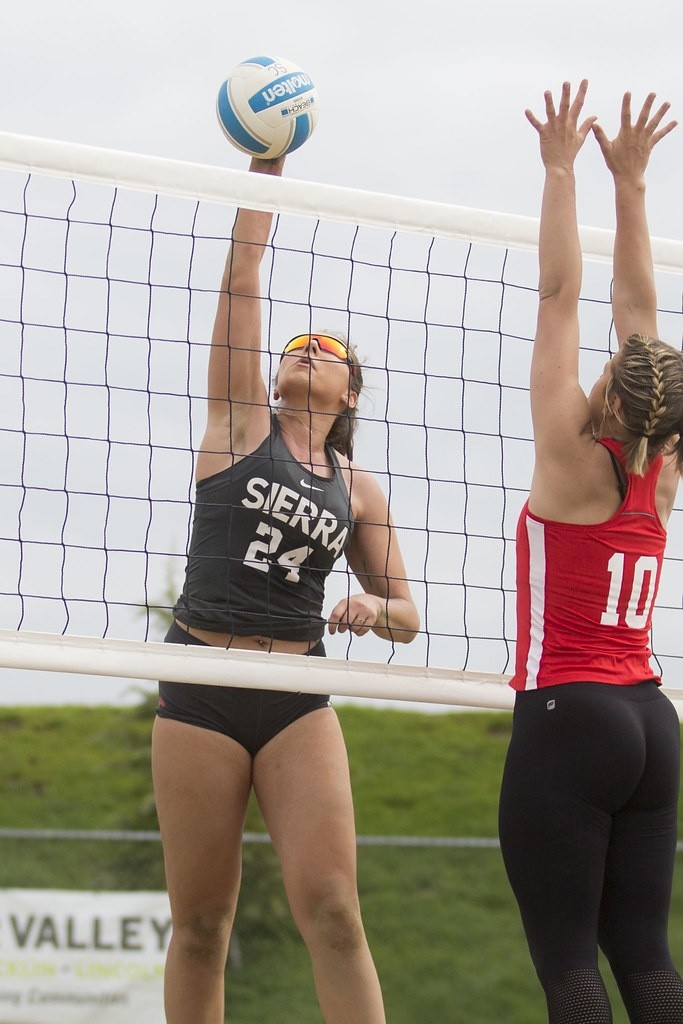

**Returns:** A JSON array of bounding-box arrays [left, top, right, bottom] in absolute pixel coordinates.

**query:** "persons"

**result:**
[[152, 154, 420, 1024], [496, 78, 682, 1022]]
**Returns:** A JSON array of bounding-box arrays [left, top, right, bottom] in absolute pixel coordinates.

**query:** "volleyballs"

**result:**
[[217, 52, 315, 163]]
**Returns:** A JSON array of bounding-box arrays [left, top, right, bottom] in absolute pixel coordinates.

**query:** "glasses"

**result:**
[[279, 334, 356, 377]]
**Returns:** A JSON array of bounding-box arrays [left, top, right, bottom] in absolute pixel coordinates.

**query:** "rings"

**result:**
[[356, 618, 365, 625]]
